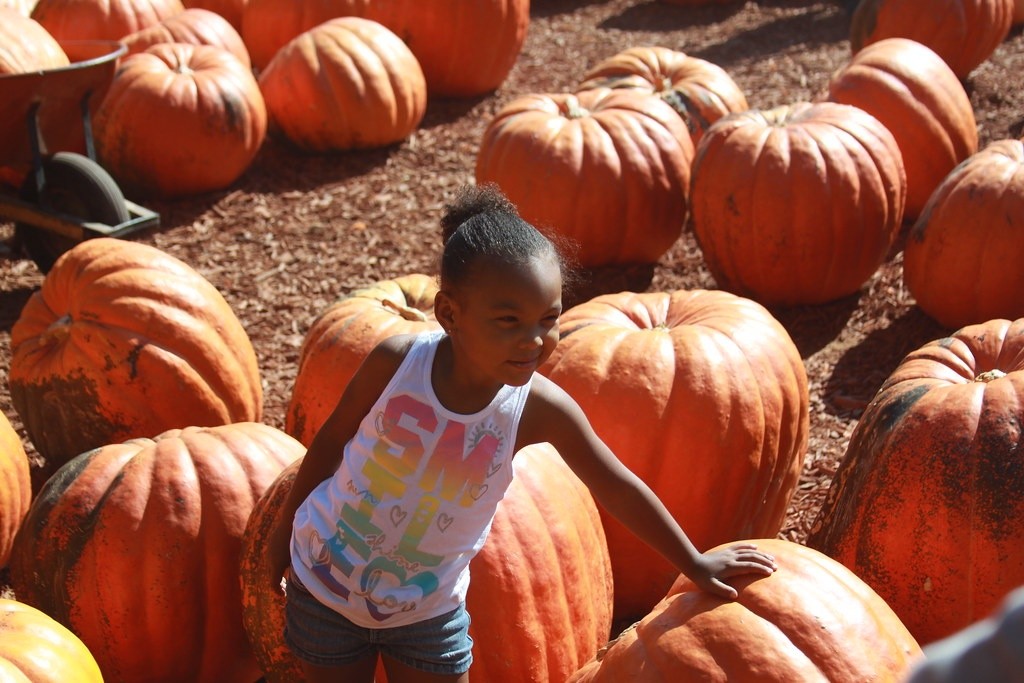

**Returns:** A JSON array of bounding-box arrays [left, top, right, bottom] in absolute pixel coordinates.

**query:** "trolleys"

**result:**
[[0, 38, 162, 277]]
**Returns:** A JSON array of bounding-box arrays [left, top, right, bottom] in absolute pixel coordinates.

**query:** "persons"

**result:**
[[268, 178, 778, 683]]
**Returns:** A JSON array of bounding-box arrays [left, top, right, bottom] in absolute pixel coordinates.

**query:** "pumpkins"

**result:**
[[0, 0, 1024, 682]]
